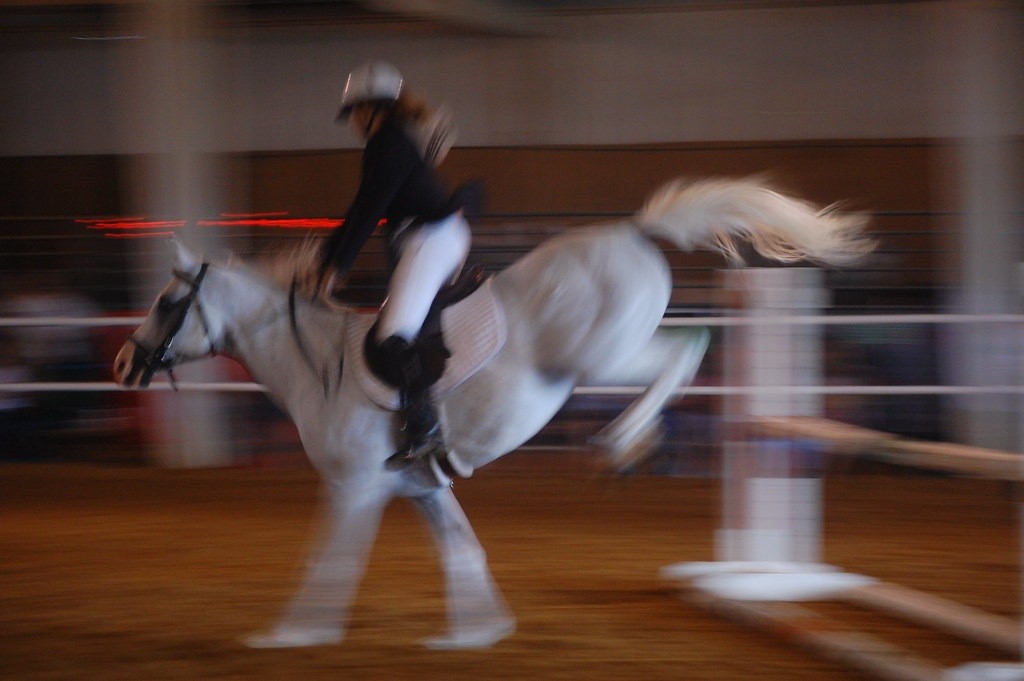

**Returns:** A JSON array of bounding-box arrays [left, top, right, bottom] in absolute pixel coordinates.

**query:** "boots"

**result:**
[[378, 334, 443, 471]]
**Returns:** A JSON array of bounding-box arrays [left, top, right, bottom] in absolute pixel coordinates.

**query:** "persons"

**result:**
[[300, 55, 486, 469]]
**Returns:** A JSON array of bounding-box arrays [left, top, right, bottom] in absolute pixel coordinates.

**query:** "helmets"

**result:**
[[334, 64, 404, 125]]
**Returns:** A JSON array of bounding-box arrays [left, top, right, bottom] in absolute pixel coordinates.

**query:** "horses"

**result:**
[[111, 171, 877, 646]]
